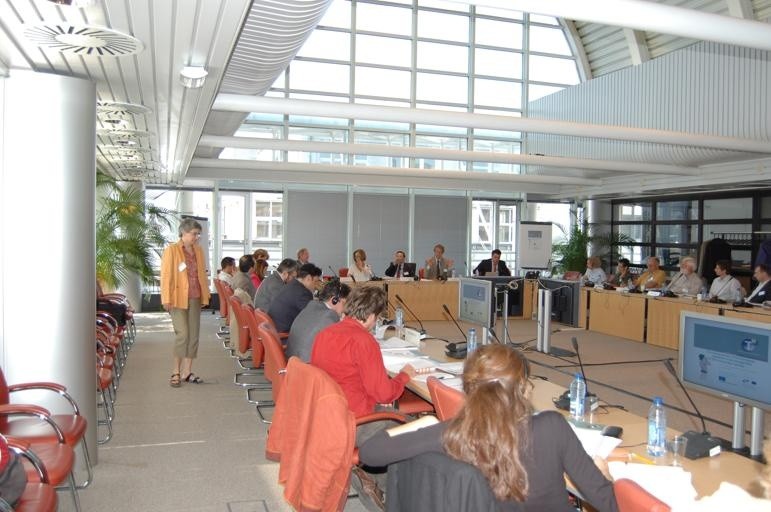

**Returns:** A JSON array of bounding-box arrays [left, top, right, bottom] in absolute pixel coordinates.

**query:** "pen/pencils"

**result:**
[[631, 452, 654, 465]]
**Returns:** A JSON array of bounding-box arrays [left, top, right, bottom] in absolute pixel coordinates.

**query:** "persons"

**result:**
[[473, 249, 511, 277], [287, 280, 350, 362], [310, 286, 418, 449], [218, 248, 322, 346], [160, 218, 211, 387], [385, 251, 414, 276], [347, 250, 373, 282], [579, 255, 771, 307], [440, 345, 616, 512], [423, 245, 454, 279]]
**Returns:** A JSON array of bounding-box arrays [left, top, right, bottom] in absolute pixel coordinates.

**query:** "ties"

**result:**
[[397, 264, 402, 278], [493, 263, 497, 272], [437, 260, 440, 280]]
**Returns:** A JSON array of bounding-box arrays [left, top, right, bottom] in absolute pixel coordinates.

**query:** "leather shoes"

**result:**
[[350, 467, 385, 512]]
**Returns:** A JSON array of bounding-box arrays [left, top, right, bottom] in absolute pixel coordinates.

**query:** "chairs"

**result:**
[[0, 440, 58, 512], [0, 367, 92, 512], [0, 409, 81, 512], [96, 280, 138, 445]]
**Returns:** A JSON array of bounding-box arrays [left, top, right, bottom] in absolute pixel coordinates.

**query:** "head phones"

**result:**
[[333, 290, 339, 306]]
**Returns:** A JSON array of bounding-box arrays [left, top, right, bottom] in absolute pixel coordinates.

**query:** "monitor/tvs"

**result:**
[[556, 334, 595, 409], [458, 276, 498, 331], [539, 277, 580, 329], [677, 309, 770, 412]]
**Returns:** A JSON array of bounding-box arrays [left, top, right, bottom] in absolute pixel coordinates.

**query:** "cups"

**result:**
[[664, 435, 687, 467], [585, 396, 599, 425]]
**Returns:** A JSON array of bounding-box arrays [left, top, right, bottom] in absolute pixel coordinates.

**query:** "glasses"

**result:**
[[189, 231, 200, 235]]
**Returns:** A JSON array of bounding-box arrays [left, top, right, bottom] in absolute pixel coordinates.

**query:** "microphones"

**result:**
[[445, 302, 469, 352], [711, 276, 733, 301], [464, 260, 470, 274], [397, 293, 427, 334], [327, 264, 337, 275], [488, 326, 501, 340], [663, 357, 722, 462]]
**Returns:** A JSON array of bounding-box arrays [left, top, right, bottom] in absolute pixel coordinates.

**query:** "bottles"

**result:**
[[395, 306, 403, 327], [569, 373, 585, 422], [467, 328, 476, 355], [647, 397, 667, 457], [596, 274, 742, 304]]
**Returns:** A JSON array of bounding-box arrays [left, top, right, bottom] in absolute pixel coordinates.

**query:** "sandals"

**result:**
[[171, 374, 203, 387]]
